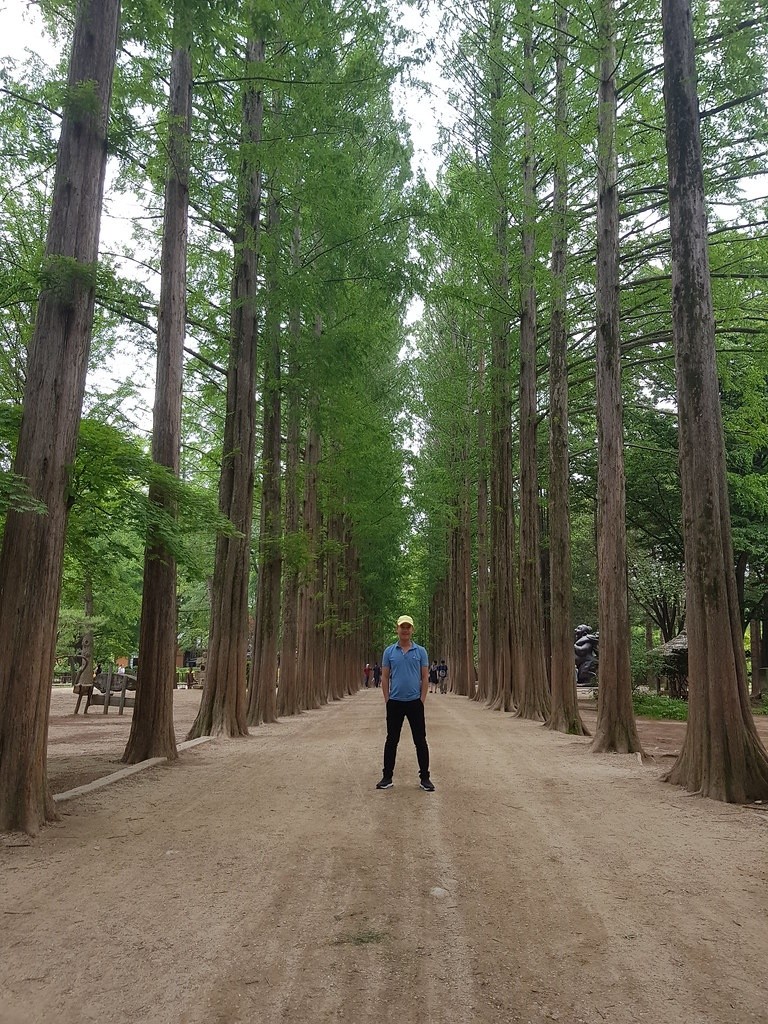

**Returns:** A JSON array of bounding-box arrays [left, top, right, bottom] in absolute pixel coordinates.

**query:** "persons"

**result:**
[[90, 658, 131, 680], [572, 624, 600, 683], [375, 615, 436, 792], [186, 667, 197, 690], [363, 658, 383, 687], [426, 659, 448, 694]]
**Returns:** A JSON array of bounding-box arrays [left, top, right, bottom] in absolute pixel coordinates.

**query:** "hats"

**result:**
[[397, 615, 414, 627]]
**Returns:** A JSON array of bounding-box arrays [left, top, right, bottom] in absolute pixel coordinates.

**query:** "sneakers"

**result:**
[[420, 780, 435, 791], [376, 778, 394, 789]]
